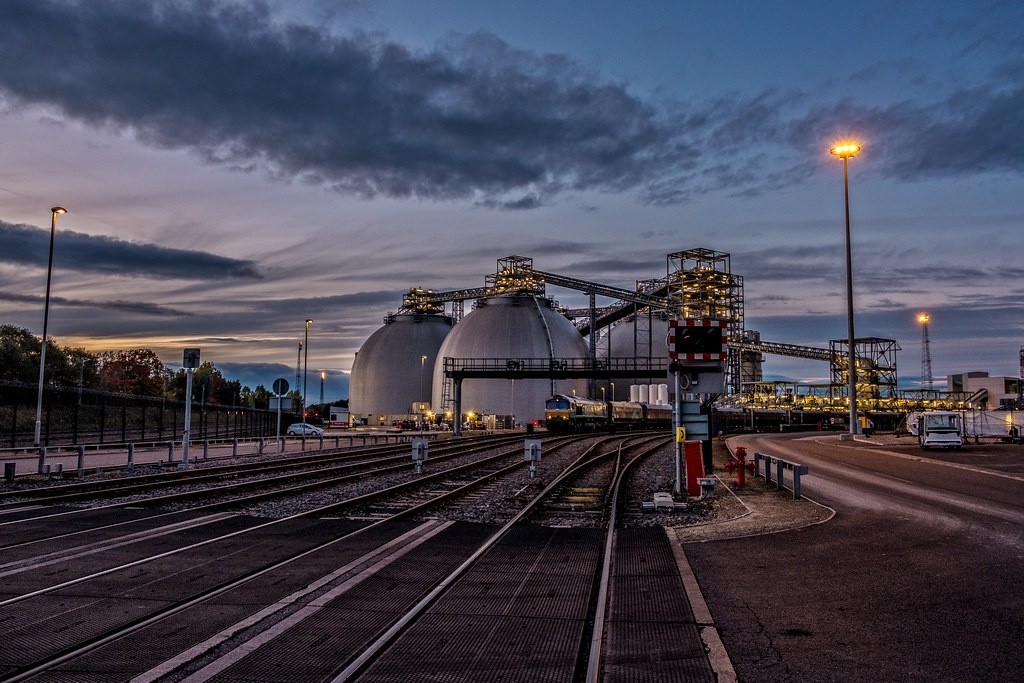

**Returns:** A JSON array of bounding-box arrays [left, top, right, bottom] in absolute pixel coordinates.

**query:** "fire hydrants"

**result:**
[[724, 446, 757, 488]]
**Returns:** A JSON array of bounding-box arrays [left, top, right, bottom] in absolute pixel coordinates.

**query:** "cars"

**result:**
[[287, 423, 324, 436]]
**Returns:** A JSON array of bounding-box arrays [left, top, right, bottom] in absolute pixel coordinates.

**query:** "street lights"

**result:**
[[601, 386, 607, 400], [610, 382, 615, 400], [420, 355, 427, 429], [34, 206, 68, 446], [829, 142, 866, 441], [302, 319, 313, 435]]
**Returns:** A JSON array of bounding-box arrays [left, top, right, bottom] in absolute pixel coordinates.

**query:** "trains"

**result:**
[[545, 394, 907, 436]]
